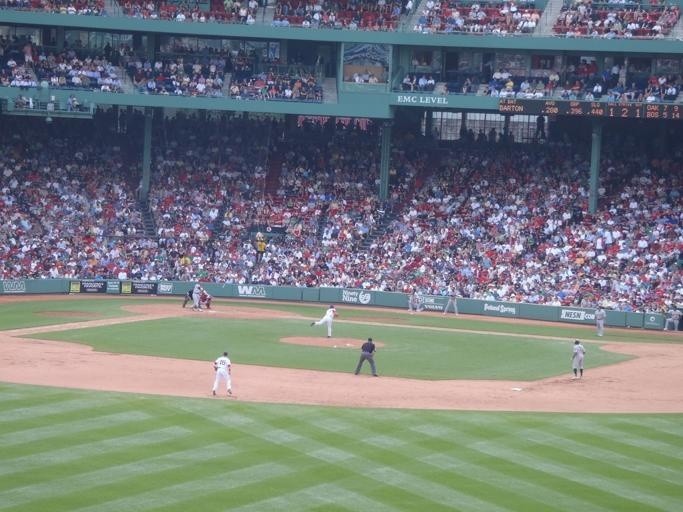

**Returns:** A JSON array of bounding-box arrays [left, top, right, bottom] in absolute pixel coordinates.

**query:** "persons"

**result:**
[[593, 305, 607, 336], [212, 350, 231, 396], [309, 304, 339, 339], [442, 286, 459, 316], [662, 306, 681, 332], [569, 340, 585, 379], [352, 338, 377, 377], [0, 1, 683, 313]]
